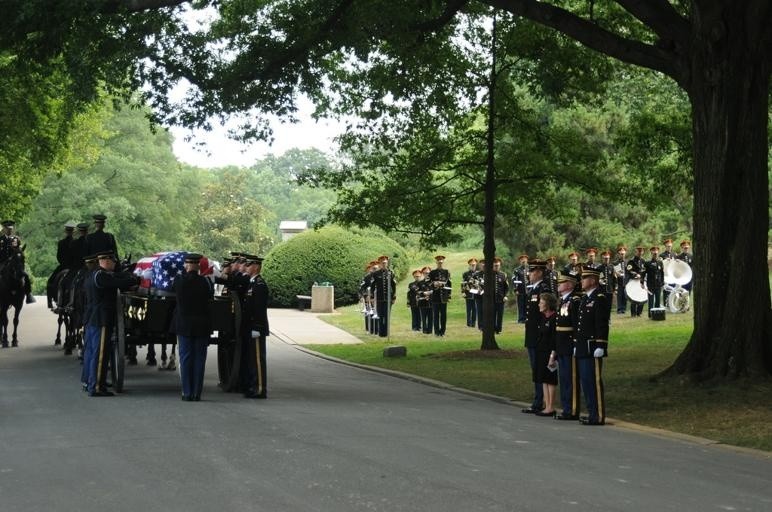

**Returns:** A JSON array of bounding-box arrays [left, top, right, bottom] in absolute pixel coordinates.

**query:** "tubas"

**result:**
[[663, 258, 692, 313]]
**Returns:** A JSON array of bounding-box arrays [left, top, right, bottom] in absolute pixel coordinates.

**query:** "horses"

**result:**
[[47, 252, 177, 370], [0, 243, 28, 348]]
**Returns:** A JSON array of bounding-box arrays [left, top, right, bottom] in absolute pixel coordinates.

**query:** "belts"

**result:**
[[556, 326, 573, 331]]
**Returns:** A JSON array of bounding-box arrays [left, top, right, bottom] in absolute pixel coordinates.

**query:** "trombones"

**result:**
[[356, 288, 380, 338]]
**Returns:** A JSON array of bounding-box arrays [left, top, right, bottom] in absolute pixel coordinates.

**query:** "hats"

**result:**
[[468, 258, 502, 267], [1, 220, 15, 229], [601, 239, 690, 259], [77, 223, 90, 230], [83, 248, 117, 263], [435, 256, 446, 263], [185, 254, 203, 263], [92, 214, 106, 222], [365, 256, 389, 271], [64, 225, 76, 232], [518, 247, 602, 284], [222, 252, 264, 267], [413, 266, 432, 279]]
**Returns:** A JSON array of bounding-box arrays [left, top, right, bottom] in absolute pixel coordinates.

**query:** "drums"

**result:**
[[650, 308, 665, 320], [625, 279, 649, 302]]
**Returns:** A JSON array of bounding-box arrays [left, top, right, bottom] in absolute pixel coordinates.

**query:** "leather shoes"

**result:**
[[26, 292, 35, 303], [522, 407, 605, 424], [89, 391, 114, 397], [244, 392, 267, 398]]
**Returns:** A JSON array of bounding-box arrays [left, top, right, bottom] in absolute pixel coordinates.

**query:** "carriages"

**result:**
[[48, 250, 245, 394]]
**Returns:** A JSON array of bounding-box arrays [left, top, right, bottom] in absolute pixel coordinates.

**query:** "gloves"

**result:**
[[251, 330, 261, 338]]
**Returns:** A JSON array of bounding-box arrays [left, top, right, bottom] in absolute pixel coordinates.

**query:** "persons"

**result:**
[[554, 269, 581, 421], [406, 255, 452, 340], [362, 255, 397, 338], [512, 255, 531, 324], [571, 265, 611, 426], [522, 261, 545, 414], [0, 220, 35, 309], [460, 256, 509, 336], [47, 213, 269, 401], [533, 240, 693, 320], [535, 292, 561, 416]]
